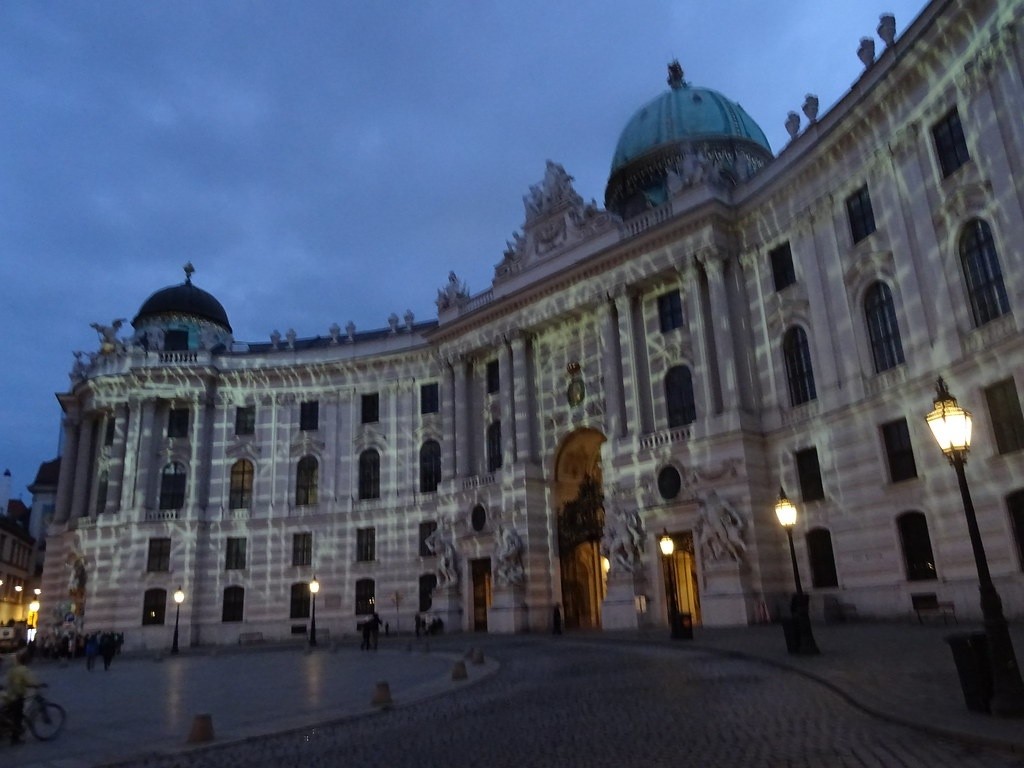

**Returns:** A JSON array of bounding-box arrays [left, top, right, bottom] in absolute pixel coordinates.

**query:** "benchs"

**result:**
[[307, 628, 330, 641], [910, 590, 959, 627], [238, 632, 264, 646]]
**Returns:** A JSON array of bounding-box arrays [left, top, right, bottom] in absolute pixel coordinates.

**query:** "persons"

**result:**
[[6, 654, 44, 745], [90, 321, 122, 344], [665, 142, 735, 195], [360, 612, 384, 652], [27, 628, 124, 672], [424, 526, 456, 586], [504, 159, 576, 260], [494, 523, 520, 586], [415, 612, 444, 636], [695, 489, 745, 564], [605, 505, 643, 580], [435, 270, 470, 312]]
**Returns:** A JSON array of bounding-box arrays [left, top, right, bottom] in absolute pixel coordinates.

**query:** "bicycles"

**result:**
[[0, 681, 67, 746]]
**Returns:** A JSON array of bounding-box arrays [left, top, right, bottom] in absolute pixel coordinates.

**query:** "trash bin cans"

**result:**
[[678, 613, 693, 638], [781, 615, 801, 655], [943, 628, 999, 712]]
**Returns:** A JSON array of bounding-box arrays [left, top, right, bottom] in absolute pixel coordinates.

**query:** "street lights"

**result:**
[[659, 525, 683, 640], [924, 372, 1023, 717], [170, 585, 185, 655], [776, 484, 820, 657], [308, 575, 319, 647]]
